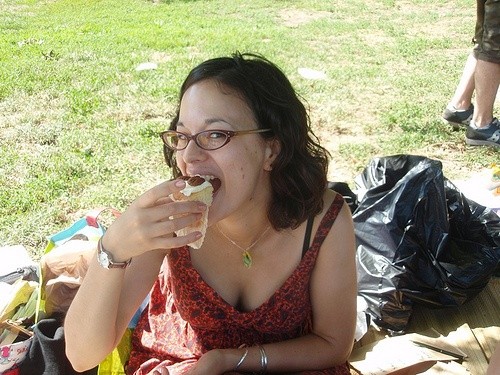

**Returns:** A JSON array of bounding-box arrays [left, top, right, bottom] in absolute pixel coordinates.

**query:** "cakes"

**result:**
[[165, 175, 214, 249]]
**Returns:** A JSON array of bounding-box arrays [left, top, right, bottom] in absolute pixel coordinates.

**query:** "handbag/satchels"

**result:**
[[36, 205, 122, 316]]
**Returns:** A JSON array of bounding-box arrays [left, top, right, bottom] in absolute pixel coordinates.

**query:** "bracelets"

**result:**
[[259, 344, 268, 375], [233, 343, 249, 371]]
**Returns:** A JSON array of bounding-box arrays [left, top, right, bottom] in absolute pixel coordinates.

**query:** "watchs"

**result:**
[[98, 235, 133, 271]]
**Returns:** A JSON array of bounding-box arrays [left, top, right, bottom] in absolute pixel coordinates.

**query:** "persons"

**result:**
[[440, 0, 500, 148], [63, 49, 358, 375]]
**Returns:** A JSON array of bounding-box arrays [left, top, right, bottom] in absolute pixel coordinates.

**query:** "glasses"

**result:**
[[160, 130, 270, 150]]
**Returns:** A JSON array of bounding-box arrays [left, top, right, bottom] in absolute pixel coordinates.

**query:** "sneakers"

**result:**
[[442, 103, 473, 128], [465, 118, 500, 148]]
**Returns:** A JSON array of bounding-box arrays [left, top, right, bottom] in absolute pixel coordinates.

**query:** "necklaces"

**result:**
[[215, 221, 272, 270]]
